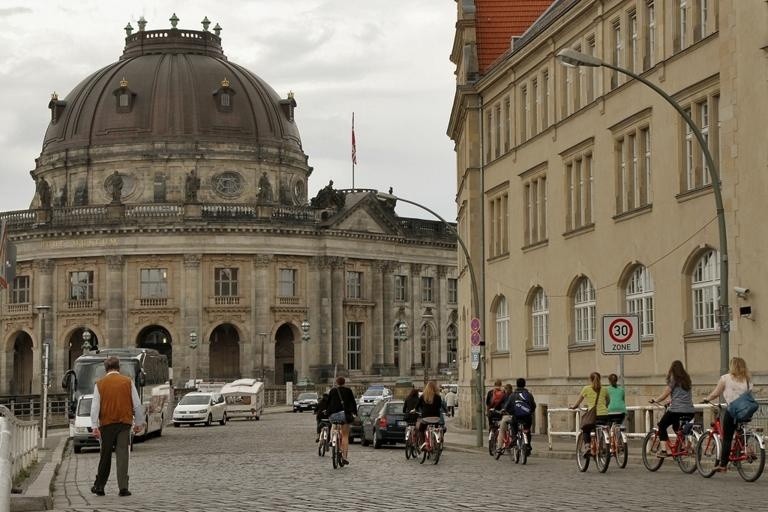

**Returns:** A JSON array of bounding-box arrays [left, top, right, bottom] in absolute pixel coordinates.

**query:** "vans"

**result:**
[[71, 393, 136, 453]]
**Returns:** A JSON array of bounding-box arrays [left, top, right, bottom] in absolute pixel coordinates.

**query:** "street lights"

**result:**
[[189, 331, 198, 392], [301, 318, 311, 380], [257, 332, 268, 384], [377, 190, 484, 448], [81, 331, 92, 356], [34, 304, 51, 437], [396, 318, 410, 379], [555, 46, 732, 439], [451, 359, 457, 383]]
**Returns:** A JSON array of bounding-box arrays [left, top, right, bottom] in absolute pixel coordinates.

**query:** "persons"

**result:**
[[402, 376, 458, 451], [485, 378, 538, 458], [321, 180, 338, 203], [257, 170, 273, 201], [599, 373, 626, 453], [567, 371, 610, 457], [36, 176, 51, 208], [315, 375, 359, 467], [90, 356, 142, 496], [704, 356, 758, 471], [650, 360, 697, 458], [109, 169, 123, 202], [185, 168, 200, 199]]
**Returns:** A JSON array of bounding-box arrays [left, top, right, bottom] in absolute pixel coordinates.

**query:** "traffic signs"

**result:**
[[600, 312, 640, 354]]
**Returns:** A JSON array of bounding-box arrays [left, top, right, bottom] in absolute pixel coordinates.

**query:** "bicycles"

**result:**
[[598, 406, 629, 468], [642, 399, 702, 474], [569, 403, 611, 473], [318, 418, 334, 457], [414, 416, 445, 465], [486, 407, 530, 465], [330, 421, 350, 469], [696, 399, 766, 482], [404, 417, 420, 461]]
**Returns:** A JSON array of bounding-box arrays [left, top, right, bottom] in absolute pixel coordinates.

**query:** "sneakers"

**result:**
[[91, 487, 106, 495], [119, 491, 131, 496]]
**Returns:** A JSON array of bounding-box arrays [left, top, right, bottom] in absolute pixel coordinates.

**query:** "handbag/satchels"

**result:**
[[329, 410, 345, 424], [581, 406, 596, 429], [729, 390, 759, 424]]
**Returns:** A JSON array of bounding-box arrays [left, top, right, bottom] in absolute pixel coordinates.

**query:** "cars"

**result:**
[[291, 392, 322, 413], [347, 399, 396, 445], [184, 379, 203, 390], [172, 391, 226, 427], [437, 383, 459, 406], [358, 385, 394, 404], [361, 400, 411, 449]]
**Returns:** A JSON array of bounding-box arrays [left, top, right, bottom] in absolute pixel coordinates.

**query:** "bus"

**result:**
[[61, 348, 170, 453], [221, 378, 265, 421]]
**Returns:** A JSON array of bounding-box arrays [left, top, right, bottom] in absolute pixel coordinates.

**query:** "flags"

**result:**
[[351, 114, 358, 165]]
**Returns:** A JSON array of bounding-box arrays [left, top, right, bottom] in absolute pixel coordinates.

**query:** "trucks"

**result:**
[[197, 377, 237, 394]]
